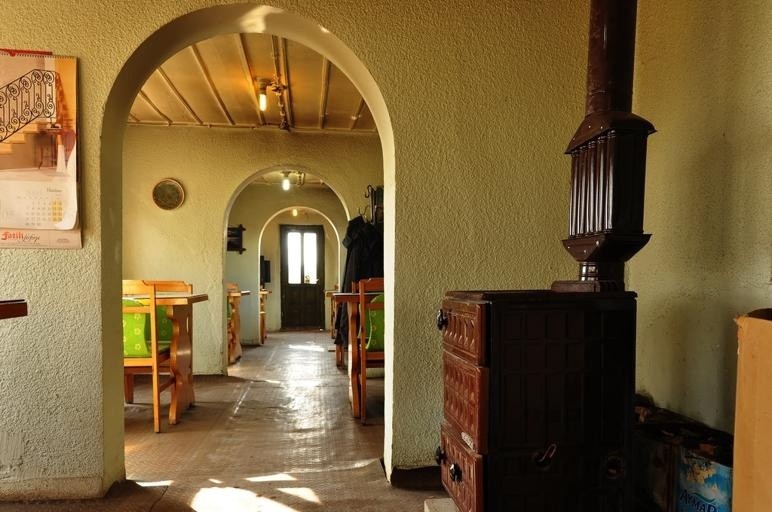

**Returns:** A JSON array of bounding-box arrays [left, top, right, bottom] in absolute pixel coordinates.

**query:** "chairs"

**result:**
[[144, 280, 193, 344], [123, 279, 174, 433], [358, 276, 384, 426]]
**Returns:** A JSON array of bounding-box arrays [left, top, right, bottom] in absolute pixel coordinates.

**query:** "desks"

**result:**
[[333, 292, 382, 419], [137, 293, 209, 426]]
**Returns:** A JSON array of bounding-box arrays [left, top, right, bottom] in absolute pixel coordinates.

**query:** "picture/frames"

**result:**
[[227, 224, 246, 254]]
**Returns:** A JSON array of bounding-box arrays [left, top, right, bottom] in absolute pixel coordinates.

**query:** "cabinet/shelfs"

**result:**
[[435, 291, 638, 512]]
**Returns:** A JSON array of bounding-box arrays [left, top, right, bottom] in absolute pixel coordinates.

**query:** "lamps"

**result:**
[[281, 171, 291, 191], [258, 79, 268, 111]]
